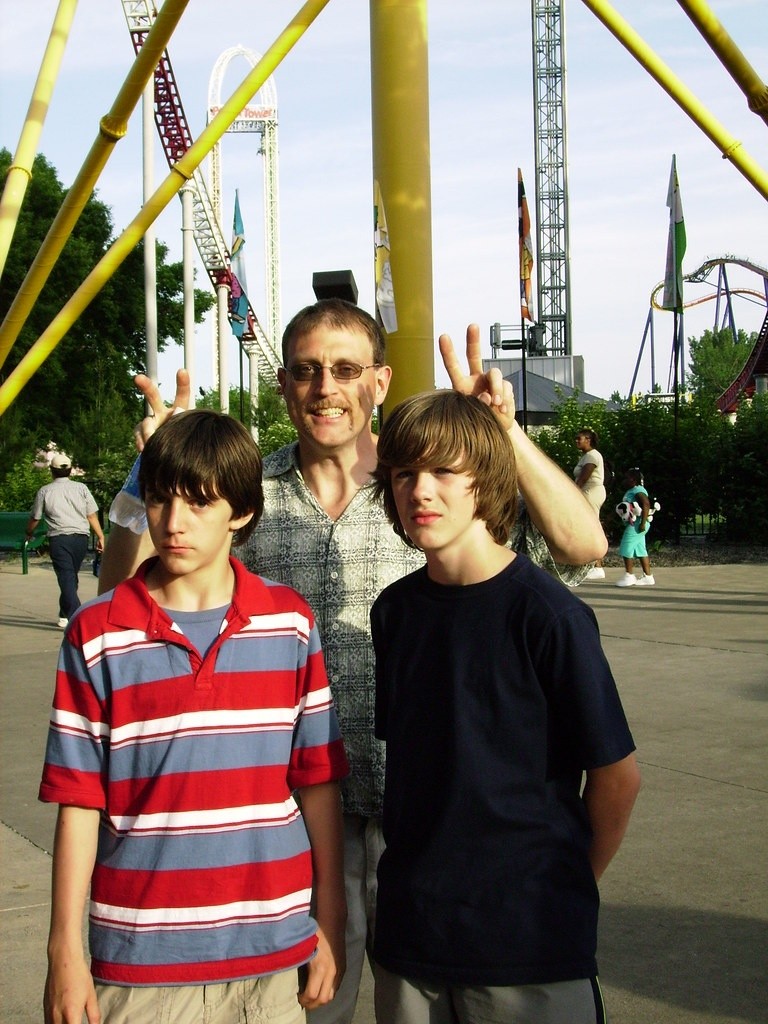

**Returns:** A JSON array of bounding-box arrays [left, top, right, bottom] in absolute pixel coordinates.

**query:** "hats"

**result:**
[[50, 455, 71, 469]]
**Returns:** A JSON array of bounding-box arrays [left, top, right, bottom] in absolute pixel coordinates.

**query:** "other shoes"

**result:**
[[57, 618, 68, 627]]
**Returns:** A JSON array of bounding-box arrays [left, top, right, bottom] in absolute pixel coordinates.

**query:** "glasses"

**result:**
[[284, 363, 380, 381]]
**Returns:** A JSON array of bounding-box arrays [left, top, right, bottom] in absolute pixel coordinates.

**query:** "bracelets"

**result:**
[[25, 529, 33, 536]]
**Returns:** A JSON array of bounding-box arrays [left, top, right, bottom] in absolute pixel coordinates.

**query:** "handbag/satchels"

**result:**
[[93, 551, 103, 576]]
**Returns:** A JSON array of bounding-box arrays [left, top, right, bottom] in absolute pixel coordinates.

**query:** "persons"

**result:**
[[614, 468, 657, 586], [371, 391, 642, 1024], [96, 302, 607, 1024], [26, 455, 104, 627], [573, 430, 607, 579], [37, 409, 352, 1024]]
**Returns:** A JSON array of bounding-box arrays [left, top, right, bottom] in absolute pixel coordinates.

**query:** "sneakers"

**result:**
[[584, 567, 606, 579], [635, 573, 655, 586], [616, 573, 637, 587]]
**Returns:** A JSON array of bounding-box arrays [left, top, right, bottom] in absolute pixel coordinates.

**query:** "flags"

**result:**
[[518, 169, 534, 321], [231, 190, 248, 338], [662, 153, 687, 313], [374, 181, 399, 333]]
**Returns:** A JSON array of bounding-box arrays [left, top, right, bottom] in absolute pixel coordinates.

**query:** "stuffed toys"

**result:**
[[617, 497, 662, 522]]
